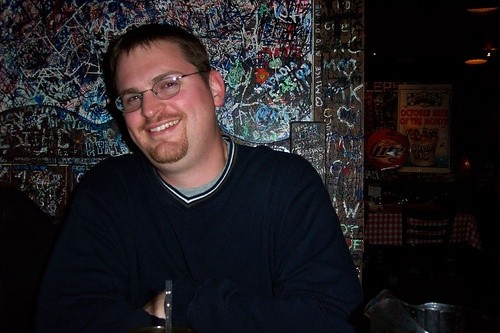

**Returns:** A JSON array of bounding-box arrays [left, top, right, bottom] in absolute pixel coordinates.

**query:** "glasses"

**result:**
[[114, 70, 204, 113]]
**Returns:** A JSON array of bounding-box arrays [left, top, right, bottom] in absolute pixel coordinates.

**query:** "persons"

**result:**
[[37, 23, 363, 333]]
